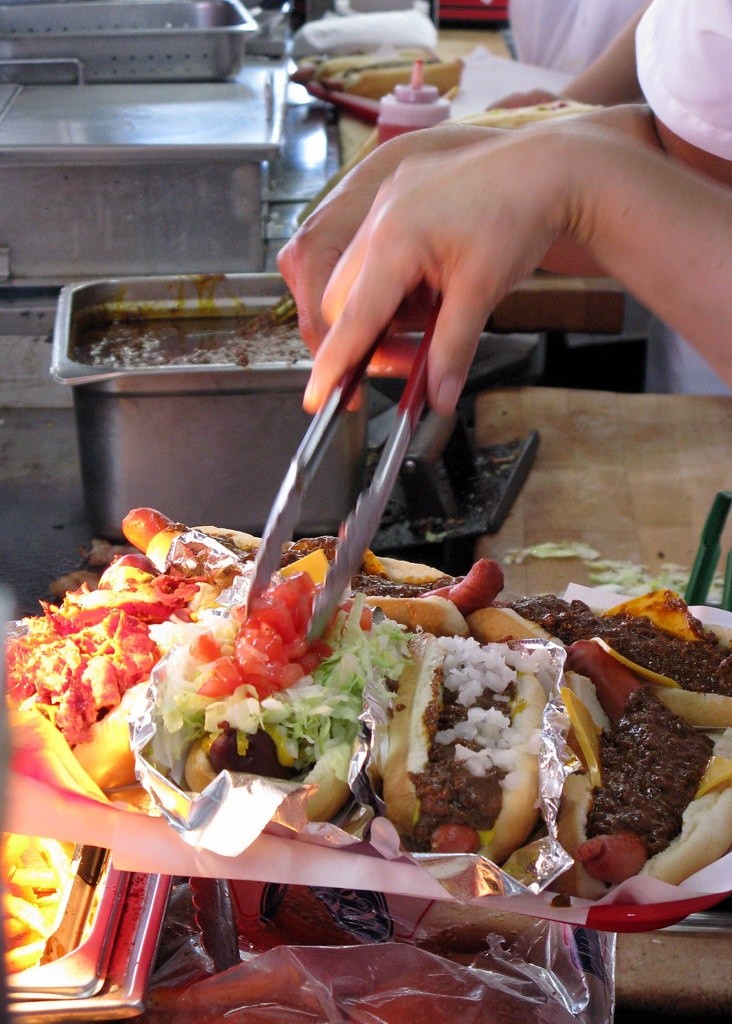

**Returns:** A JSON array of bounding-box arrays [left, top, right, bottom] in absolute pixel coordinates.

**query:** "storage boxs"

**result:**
[[48, 266, 369, 537], [0, 0, 262, 87]]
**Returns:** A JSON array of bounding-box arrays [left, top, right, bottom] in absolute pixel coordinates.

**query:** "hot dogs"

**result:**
[[290, 45, 605, 128], [5, 508, 732, 901]]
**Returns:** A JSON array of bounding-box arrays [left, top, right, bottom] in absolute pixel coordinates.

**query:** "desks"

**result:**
[[439, 383, 732, 1009], [335, 25, 626, 391]]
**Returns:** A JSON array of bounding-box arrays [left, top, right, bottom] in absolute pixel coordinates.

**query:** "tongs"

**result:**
[[245, 289, 443, 643], [36, 842, 243, 974]]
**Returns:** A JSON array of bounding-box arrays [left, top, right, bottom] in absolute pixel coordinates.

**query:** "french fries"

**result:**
[[4, 836, 77, 969]]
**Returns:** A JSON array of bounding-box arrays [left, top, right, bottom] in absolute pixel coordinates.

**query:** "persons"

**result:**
[[278, 0, 732, 416]]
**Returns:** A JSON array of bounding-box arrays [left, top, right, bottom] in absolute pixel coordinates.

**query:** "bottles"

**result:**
[[377, 57, 451, 147]]
